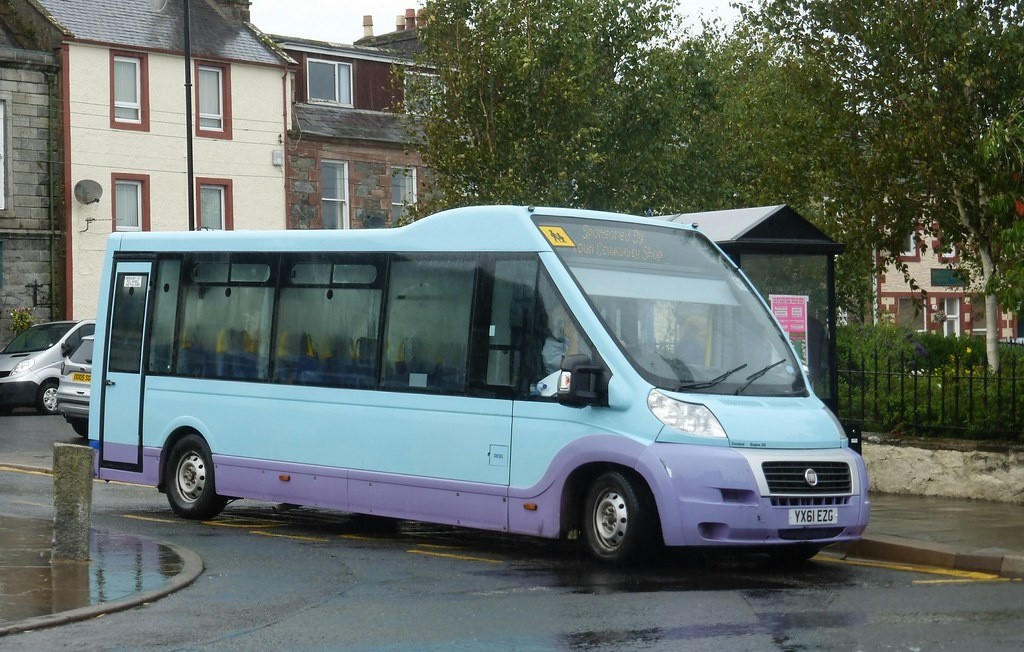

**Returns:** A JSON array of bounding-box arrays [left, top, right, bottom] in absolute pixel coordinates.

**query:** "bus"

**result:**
[[87, 204, 872, 570]]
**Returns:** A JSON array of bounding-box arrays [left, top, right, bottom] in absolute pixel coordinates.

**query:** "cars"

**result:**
[[55, 334, 94, 439]]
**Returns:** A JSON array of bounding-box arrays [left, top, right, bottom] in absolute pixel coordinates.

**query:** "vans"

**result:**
[[0, 319, 96, 415]]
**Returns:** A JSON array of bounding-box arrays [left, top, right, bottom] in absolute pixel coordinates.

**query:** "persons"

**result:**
[[540, 305, 627, 389]]
